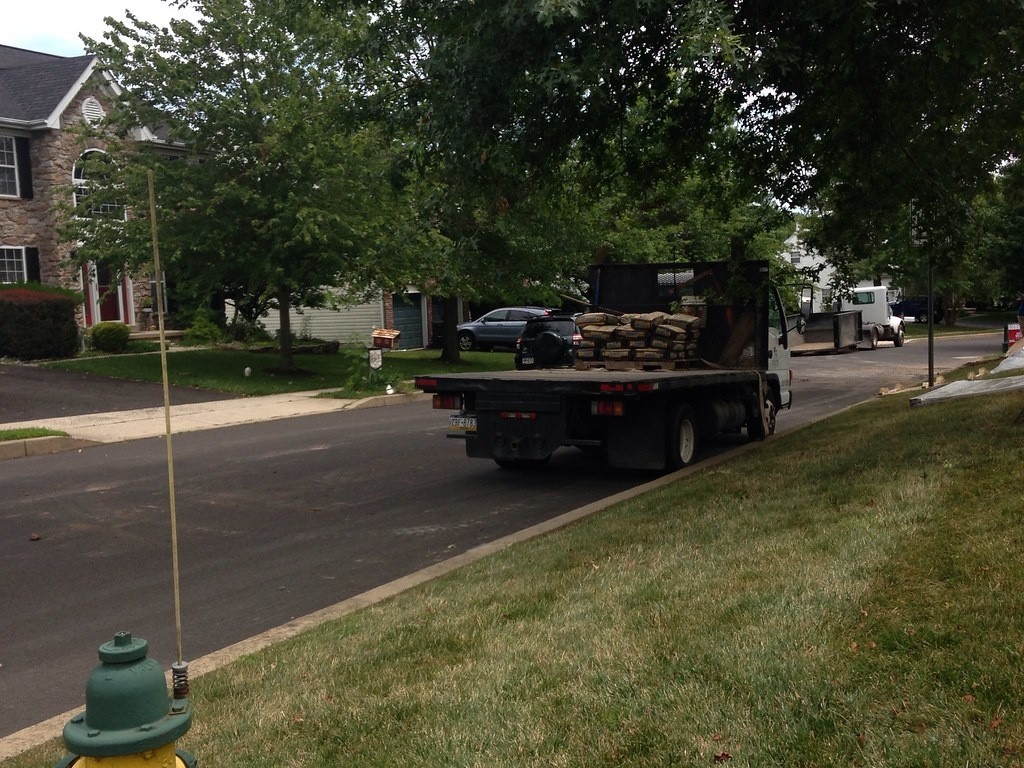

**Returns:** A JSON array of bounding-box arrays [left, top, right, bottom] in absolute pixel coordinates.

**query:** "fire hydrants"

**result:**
[[55, 630, 200, 768]]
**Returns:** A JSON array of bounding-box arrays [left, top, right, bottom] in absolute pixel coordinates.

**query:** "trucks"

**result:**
[[833, 286, 905, 351], [414, 261, 808, 480]]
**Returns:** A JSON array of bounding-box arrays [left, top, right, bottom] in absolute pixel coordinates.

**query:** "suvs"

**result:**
[[514, 311, 584, 370], [456, 305, 559, 351], [888, 294, 944, 325]]
